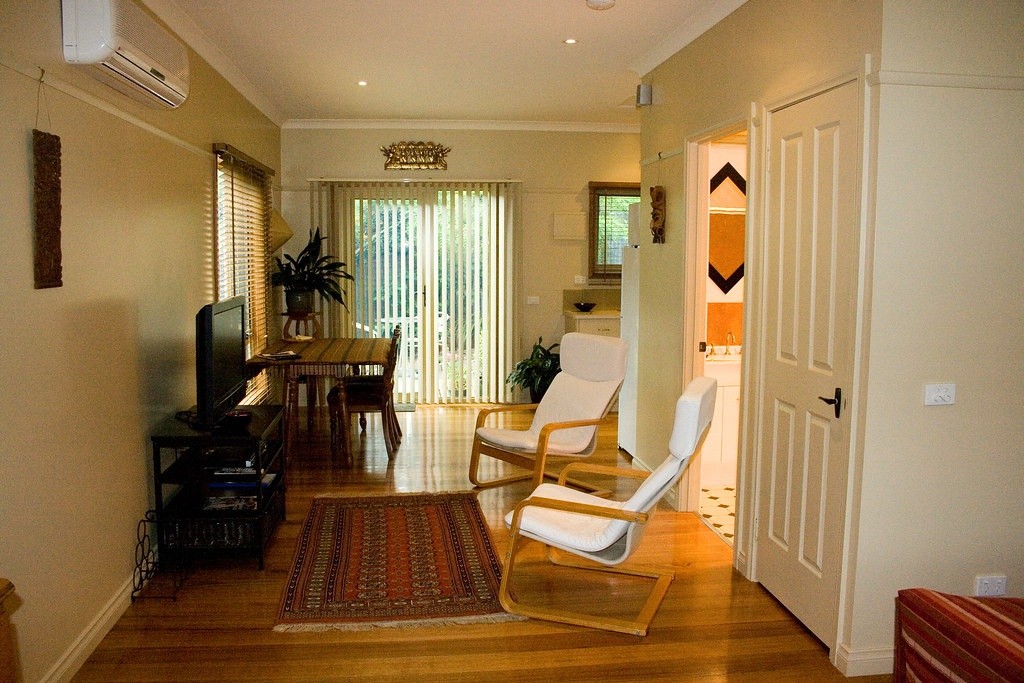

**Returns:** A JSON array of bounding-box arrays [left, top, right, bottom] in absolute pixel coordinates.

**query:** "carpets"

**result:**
[[273, 491, 529, 631]]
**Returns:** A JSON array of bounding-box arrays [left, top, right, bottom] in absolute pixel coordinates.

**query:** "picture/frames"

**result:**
[[552, 211, 589, 240]]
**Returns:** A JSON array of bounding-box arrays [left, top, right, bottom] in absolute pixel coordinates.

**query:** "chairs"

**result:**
[[500, 374, 718, 637], [326, 321, 403, 461], [468, 330, 628, 501]]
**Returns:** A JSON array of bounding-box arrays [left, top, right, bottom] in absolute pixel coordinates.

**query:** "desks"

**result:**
[[148, 405, 289, 572], [250, 337, 391, 467]]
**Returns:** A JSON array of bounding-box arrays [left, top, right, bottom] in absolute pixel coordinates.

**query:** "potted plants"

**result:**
[[506, 337, 562, 413], [271, 231, 355, 313]]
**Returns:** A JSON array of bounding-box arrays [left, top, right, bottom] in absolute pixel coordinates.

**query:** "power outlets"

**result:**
[[976, 576, 1007, 597]]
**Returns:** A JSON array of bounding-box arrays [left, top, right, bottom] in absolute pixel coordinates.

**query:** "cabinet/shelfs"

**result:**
[[564, 316, 621, 337]]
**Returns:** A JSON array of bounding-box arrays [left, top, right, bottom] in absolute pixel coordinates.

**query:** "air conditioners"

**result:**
[[60, 0, 192, 111]]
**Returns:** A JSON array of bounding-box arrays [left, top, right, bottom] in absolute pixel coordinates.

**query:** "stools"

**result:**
[[283, 314, 326, 439]]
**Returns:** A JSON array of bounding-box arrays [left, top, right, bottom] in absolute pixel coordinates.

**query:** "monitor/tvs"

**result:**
[[188, 295, 247, 434]]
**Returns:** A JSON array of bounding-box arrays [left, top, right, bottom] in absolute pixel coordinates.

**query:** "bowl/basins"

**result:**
[[573, 302, 597, 312]]
